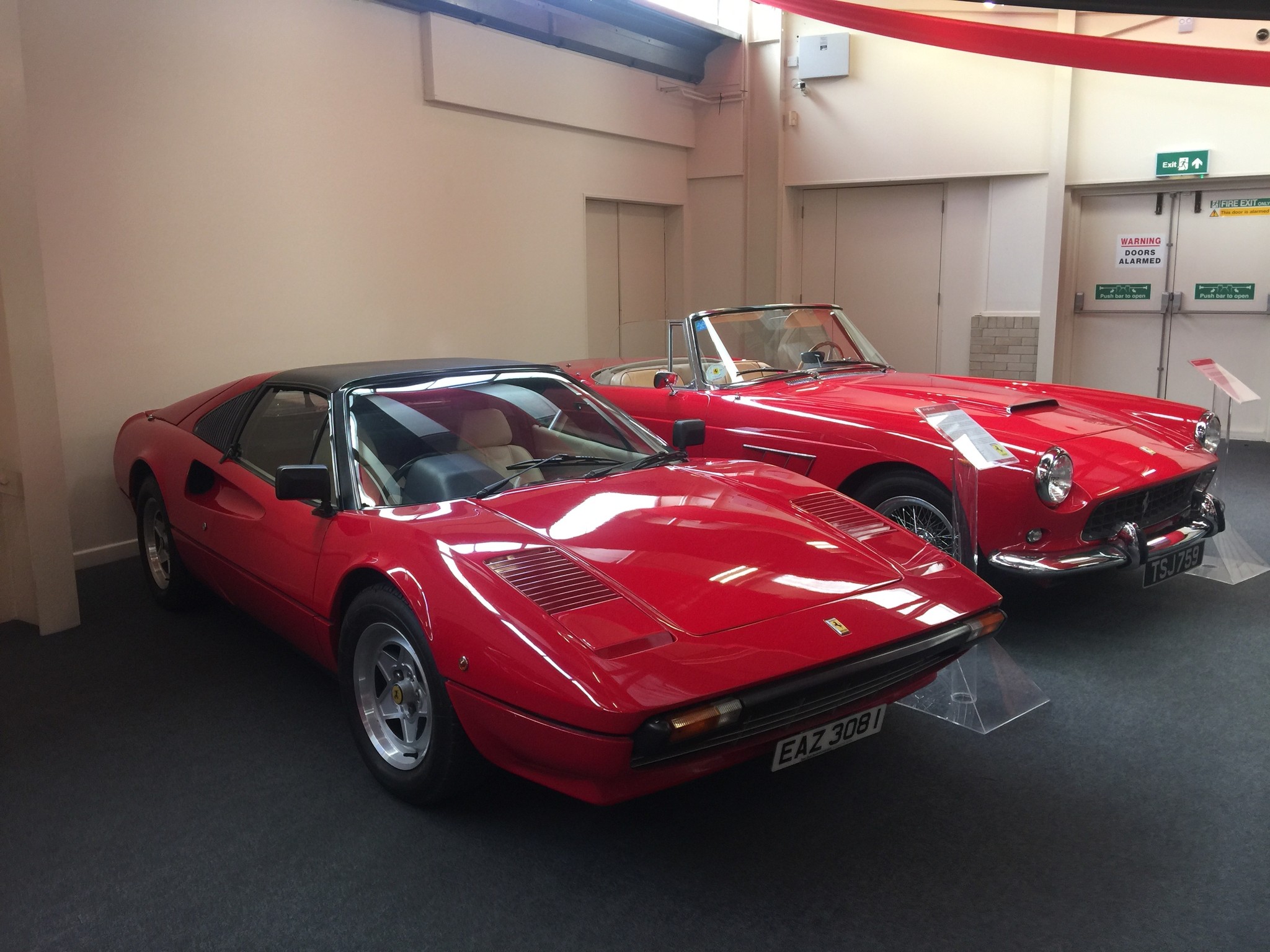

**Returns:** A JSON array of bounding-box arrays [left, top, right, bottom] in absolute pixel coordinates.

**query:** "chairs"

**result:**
[[452, 408, 545, 489], [707, 360, 778, 385], [609, 367, 685, 389], [312, 426, 407, 508]]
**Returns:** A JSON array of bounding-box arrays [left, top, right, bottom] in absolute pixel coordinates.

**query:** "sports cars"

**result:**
[[543, 303, 1226, 613], [114, 359, 1012, 813]]
